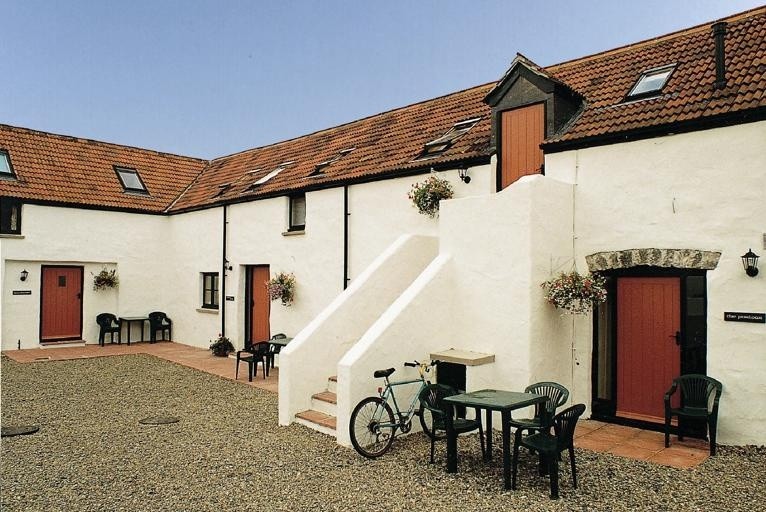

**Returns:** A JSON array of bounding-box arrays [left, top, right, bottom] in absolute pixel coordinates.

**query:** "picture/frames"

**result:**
[[215, 350, 227, 357]]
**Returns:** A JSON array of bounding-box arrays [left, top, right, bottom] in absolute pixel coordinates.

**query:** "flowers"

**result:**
[[539, 259, 609, 315], [263, 270, 297, 307], [406, 167, 454, 220], [90, 265, 119, 293], [208, 332, 234, 354]]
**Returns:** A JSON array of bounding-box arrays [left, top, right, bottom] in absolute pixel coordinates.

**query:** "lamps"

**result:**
[[19, 268, 28, 281], [741, 247, 761, 278], [456, 162, 472, 184]]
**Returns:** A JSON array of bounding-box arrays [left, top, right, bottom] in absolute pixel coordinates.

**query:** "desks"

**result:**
[[267, 337, 294, 377], [442, 388, 551, 491], [118, 316, 151, 345]]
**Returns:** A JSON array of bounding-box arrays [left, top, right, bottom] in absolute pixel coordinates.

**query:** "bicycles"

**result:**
[[349, 360, 464, 459]]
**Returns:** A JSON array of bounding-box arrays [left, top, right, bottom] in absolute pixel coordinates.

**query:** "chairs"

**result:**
[[236, 340, 269, 383], [418, 383, 485, 462], [265, 333, 286, 369], [96, 313, 121, 347], [508, 381, 570, 477], [512, 403, 588, 500], [663, 373, 724, 458], [148, 311, 172, 344]]
